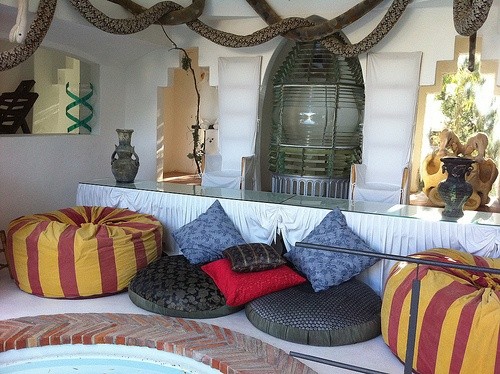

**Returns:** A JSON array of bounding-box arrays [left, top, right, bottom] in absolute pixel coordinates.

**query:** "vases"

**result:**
[[110, 129, 140, 183], [437, 158, 476, 217]]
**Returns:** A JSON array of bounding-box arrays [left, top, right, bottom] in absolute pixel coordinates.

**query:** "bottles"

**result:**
[[437, 157, 476, 218], [110, 129, 140, 183]]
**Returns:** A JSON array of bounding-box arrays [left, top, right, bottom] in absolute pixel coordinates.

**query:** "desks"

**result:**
[[75, 180, 500, 298]]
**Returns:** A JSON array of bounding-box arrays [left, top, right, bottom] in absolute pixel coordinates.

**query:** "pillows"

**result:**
[[172, 200, 384, 307]]
